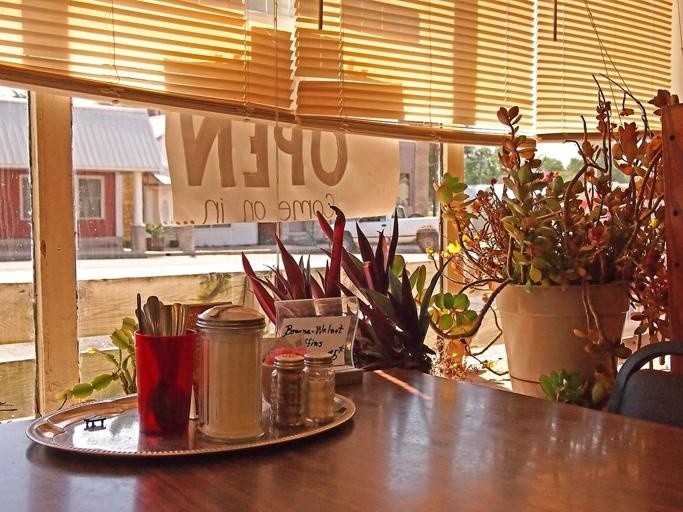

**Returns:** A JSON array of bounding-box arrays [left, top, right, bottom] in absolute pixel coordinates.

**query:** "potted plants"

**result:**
[[391, 71, 675, 402]]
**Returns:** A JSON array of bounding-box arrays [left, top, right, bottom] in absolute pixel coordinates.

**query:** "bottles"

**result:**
[[269, 353, 305, 426], [195, 304, 267, 441], [302, 351, 335, 426]]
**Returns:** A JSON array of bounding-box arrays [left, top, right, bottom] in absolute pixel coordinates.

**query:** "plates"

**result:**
[[23, 387, 357, 460]]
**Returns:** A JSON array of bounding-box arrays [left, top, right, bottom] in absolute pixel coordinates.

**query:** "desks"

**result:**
[[2, 365, 682, 510]]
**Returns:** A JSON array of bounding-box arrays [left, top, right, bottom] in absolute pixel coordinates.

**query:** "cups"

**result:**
[[133, 329, 196, 436]]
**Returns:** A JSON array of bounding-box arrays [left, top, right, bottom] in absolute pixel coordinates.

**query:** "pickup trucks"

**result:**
[[323, 203, 440, 254]]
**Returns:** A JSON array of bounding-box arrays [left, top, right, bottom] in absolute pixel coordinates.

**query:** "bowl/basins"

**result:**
[[262, 362, 275, 405]]
